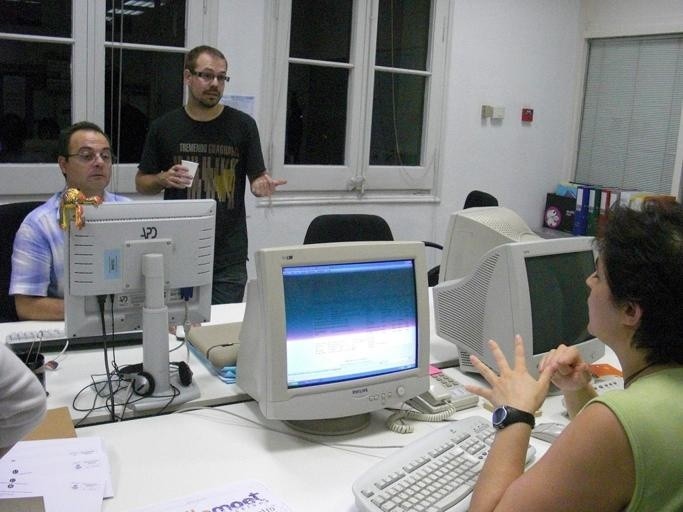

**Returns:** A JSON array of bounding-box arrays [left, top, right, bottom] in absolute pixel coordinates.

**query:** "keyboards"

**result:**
[[6, 328, 142, 350], [352, 415, 536, 512]]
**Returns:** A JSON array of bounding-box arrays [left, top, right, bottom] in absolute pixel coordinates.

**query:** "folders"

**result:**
[[574, 184, 676, 236]]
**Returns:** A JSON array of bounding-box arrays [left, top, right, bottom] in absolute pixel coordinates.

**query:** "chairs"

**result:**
[[303, 214, 394, 245], [0, 201, 46, 322], [421, 190, 498, 286]]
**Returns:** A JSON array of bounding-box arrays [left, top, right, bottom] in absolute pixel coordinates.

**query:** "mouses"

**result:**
[[531, 423, 567, 443]]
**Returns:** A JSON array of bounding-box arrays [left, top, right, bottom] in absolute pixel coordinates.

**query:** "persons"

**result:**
[[135, 45, 287, 305], [466, 193, 683, 512], [9, 120, 134, 321], [0, 343, 48, 463]]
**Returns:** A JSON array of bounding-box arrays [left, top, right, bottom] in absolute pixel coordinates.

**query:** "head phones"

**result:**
[[119, 360, 193, 396]]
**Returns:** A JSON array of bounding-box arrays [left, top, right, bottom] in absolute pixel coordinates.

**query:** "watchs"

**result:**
[[491, 405, 538, 430]]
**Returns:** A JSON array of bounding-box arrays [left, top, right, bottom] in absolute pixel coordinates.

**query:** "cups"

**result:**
[[180, 159, 199, 188]]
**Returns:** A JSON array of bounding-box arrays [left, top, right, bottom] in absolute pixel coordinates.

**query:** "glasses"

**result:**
[[69, 150, 112, 164], [189, 69, 230, 84]]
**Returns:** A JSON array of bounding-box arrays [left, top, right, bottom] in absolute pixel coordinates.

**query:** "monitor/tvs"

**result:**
[[432, 235, 606, 396], [235, 240, 432, 437], [439, 207, 545, 283], [64, 199, 217, 391]]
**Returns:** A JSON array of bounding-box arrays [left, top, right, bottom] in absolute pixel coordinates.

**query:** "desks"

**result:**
[[2, 284, 626, 512]]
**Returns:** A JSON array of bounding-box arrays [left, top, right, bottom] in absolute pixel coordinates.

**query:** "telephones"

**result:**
[[406, 365, 479, 415]]
